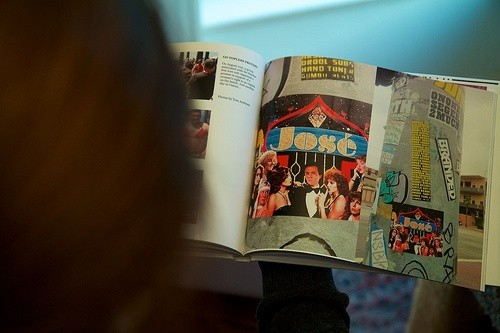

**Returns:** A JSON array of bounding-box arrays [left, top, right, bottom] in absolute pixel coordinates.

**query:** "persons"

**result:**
[[388, 224, 443, 257], [0, 0, 350, 333], [176, 58, 217, 100], [182, 109, 209, 159], [247, 150, 366, 221]]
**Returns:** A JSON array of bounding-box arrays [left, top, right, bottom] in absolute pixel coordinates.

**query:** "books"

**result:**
[[168, 41, 500, 292]]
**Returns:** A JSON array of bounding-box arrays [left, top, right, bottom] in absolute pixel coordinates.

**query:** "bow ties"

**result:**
[[308, 188, 319, 194], [415, 244, 420, 245]]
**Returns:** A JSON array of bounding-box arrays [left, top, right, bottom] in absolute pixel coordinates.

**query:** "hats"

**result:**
[[202, 59, 216, 68]]
